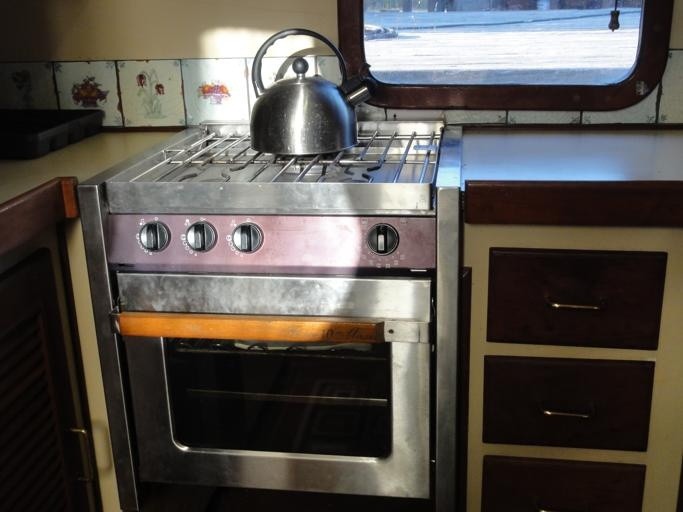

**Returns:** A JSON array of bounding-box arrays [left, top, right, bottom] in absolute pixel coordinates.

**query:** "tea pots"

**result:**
[[250, 28, 368, 154]]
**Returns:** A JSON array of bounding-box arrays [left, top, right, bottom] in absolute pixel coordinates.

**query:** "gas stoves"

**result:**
[[108, 120, 443, 209]]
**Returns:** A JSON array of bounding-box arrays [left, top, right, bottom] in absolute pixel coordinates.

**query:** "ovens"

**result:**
[[104, 215, 438, 507]]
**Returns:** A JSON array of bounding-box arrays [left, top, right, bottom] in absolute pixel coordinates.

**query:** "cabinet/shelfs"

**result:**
[[477, 245, 669, 511], [0, 245, 104, 512]]
